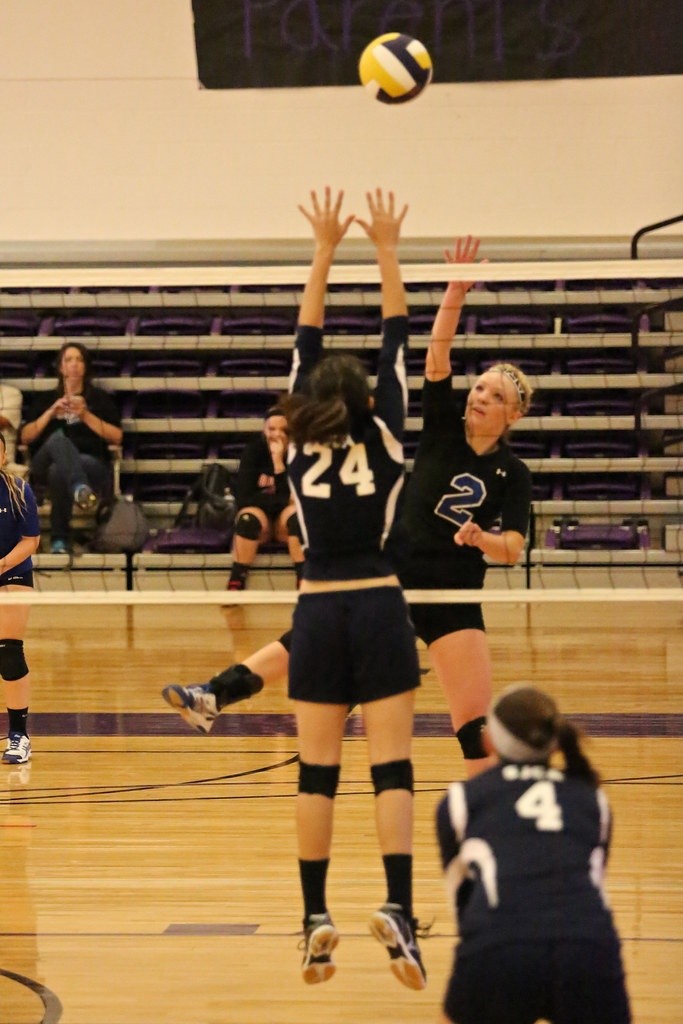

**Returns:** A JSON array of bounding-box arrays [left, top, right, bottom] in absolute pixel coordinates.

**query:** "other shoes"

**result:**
[[50, 538, 69, 555], [73, 482, 96, 509], [223, 580, 245, 609]]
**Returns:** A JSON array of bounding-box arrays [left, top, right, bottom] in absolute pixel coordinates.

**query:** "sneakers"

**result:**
[[161, 684, 223, 735], [298, 911, 339, 984], [369, 904, 435, 990], [1, 732, 31, 764]]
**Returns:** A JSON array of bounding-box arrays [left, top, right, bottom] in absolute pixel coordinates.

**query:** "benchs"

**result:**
[[0, 242, 683, 571]]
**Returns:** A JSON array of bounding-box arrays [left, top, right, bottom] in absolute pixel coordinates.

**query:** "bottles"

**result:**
[[223, 488, 235, 506]]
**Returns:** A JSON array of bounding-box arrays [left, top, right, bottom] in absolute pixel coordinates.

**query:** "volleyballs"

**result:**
[[356, 31, 435, 107]]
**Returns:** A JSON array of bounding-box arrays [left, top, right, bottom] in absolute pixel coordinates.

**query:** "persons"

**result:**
[[20, 342, 123, 554], [0, 432, 41, 764], [159, 186, 535, 991], [436, 687, 632, 1024]]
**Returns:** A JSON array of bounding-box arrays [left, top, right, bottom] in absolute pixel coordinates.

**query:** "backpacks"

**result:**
[[177, 463, 239, 527], [93, 496, 148, 552]]
